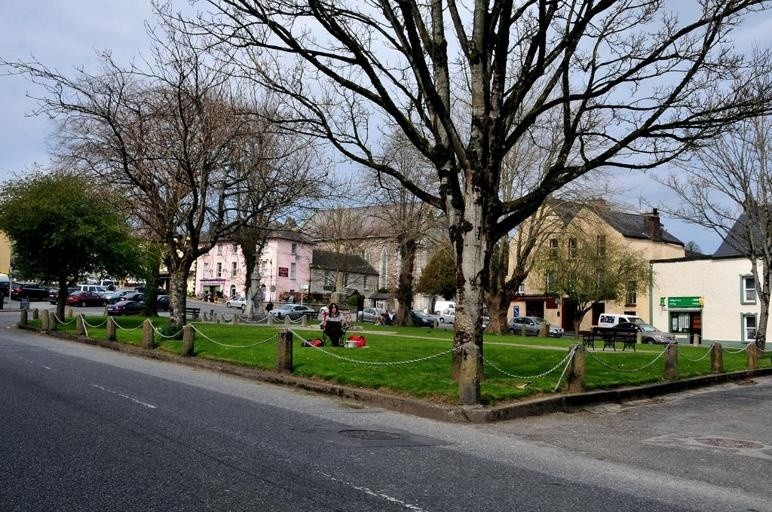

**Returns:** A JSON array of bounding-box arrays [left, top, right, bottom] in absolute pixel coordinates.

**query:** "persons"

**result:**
[[374, 309, 389, 326], [319, 303, 349, 330]]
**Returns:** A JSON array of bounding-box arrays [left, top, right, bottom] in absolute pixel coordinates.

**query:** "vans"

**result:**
[[597, 313, 645, 328], [434, 300, 456, 315]]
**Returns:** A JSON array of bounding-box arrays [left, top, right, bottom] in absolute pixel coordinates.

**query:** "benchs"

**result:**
[[185, 307, 201, 319], [579, 326, 638, 351], [294, 310, 321, 319]]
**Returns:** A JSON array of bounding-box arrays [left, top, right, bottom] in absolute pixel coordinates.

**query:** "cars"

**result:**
[[507, 316, 564, 338], [269, 303, 315, 320], [0, 282, 169, 315], [439, 308, 455, 323], [393, 309, 438, 328], [358, 307, 392, 323], [226, 296, 246, 311], [612, 322, 677, 345]]
